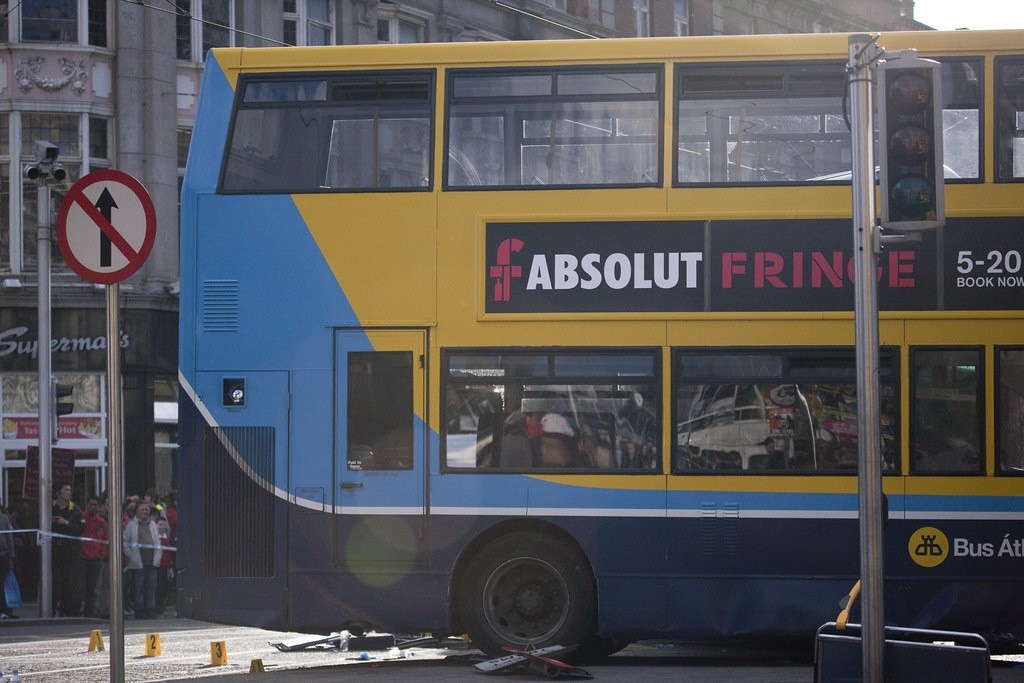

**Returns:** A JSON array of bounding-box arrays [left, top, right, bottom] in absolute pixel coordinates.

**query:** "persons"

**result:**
[[499, 412, 792, 474], [0, 481, 176, 619]]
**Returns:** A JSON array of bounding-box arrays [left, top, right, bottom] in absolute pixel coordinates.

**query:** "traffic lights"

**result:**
[[876, 51, 945, 230]]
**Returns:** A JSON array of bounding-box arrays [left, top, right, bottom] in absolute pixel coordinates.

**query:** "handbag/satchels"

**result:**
[[4, 570, 21, 608]]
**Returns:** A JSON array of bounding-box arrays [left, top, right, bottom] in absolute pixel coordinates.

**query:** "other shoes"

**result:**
[[87, 608, 99, 618], [0, 612, 7, 619], [135, 608, 158, 619], [58, 608, 77, 617], [7, 614, 19, 619]]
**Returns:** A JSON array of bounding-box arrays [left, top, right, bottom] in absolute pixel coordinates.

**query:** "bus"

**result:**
[[171, 29, 1024, 662]]
